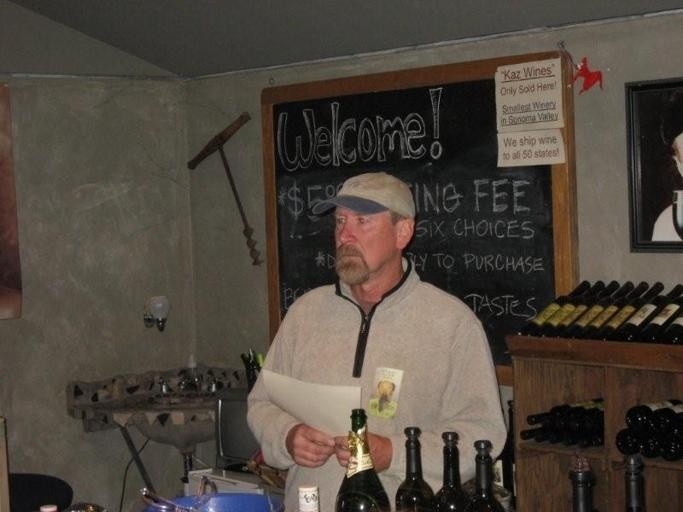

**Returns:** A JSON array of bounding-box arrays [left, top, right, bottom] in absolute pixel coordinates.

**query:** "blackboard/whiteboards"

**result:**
[[260, 50, 579, 387]]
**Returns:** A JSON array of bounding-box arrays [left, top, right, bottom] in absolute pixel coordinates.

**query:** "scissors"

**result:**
[[139, 477, 217, 512]]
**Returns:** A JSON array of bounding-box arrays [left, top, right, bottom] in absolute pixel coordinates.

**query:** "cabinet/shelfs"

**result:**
[[188, 468, 264, 496], [503, 333, 683, 512]]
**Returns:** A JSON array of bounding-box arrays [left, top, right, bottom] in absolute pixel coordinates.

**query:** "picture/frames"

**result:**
[[624, 76, 682, 254]]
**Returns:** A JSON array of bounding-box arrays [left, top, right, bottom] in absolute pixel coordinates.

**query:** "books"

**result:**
[[258, 368, 362, 440]]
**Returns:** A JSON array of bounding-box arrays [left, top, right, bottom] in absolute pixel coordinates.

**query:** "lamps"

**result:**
[[144, 297, 170, 331]]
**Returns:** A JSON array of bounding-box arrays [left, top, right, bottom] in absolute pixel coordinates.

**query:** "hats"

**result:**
[[312, 172, 416, 220]]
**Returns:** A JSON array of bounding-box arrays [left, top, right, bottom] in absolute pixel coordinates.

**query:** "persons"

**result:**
[[245, 170, 510, 509], [367, 379, 397, 416]]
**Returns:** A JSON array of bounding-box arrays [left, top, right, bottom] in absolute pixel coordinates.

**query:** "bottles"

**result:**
[[521, 400, 604, 448], [683, 309, 683, 311], [619, 397, 683, 460], [568, 470, 595, 512], [624, 455, 647, 512], [523, 281, 683, 344], [335, 409, 506, 511]]
